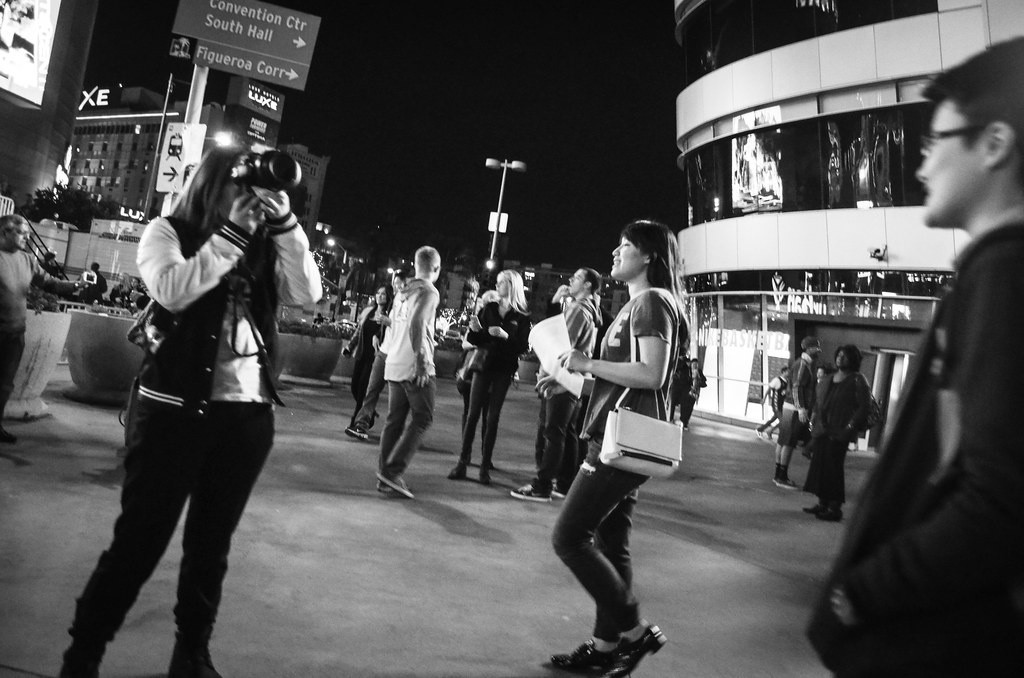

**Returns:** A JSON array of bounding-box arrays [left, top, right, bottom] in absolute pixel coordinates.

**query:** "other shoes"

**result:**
[[376, 470, 414, 499], [803, 504, 843, 521], [447, 465, 466, 478], [345, 425, 369, 440], [0, 423, 17, 444], [756, 428, 773, 440], [479, 464, 491, 485]]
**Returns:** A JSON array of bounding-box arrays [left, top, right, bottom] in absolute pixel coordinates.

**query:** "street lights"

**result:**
[[327, 238, 347, 274], [484, 157, 527, 260]]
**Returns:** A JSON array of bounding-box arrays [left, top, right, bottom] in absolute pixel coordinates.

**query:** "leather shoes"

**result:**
[[550, 623, 667, 678]]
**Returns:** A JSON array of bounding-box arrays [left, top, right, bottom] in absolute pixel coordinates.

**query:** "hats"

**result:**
[[834, 343, 861, 367], [801, 336, 820, 350]]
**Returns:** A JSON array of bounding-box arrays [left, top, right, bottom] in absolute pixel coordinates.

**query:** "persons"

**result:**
[[58, 145, 325, 678], [807, 36, 1024, 678], [0, 215, 153, 442], [335, 247, 867, 522], [535, 221, 695, 678]]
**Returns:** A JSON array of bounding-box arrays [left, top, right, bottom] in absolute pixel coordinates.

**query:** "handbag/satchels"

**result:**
[[854, 373, 883, 429], [600, 407, 683, 477]]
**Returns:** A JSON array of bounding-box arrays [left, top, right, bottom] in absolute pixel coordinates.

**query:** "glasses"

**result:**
[[921, 125, 988, 153]]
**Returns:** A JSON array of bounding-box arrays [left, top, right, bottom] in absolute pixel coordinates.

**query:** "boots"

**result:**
[[772, 463, 798, 490]]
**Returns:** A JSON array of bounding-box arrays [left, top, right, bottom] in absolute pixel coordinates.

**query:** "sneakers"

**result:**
[[511, 483, 565, 503]]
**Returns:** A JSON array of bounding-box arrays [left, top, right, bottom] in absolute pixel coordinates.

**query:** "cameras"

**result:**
[[231, 149, 302, 199]]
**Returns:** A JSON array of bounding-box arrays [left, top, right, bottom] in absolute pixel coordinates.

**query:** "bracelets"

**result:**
[[848, 424, 854, 431]]
